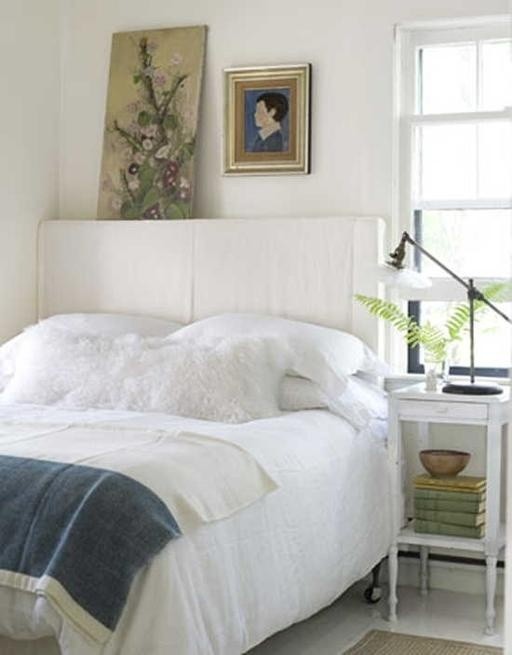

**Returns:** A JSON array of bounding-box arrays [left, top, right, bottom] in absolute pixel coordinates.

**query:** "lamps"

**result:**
[[382, 230, 511, 395]]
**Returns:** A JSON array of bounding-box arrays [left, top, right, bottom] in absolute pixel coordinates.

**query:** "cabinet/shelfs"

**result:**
[[388, 378, 511, 635]]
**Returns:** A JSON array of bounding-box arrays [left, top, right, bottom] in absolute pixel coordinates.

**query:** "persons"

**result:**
[[251, 91, 288, 152]]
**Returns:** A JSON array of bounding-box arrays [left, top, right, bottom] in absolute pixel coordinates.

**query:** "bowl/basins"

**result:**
[[418, 448, 471, 479]]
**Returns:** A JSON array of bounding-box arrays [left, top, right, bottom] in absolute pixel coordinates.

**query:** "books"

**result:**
[[412, 474, 486, 541]]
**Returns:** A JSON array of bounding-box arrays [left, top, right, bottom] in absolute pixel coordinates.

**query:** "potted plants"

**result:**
[[340, 276, 511, 390]]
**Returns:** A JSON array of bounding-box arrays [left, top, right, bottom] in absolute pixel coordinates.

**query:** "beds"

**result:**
[[0, 214, 390, 653]]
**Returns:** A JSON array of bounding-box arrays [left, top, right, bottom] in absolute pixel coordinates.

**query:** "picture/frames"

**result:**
[[220, 60, 315, 178]]
